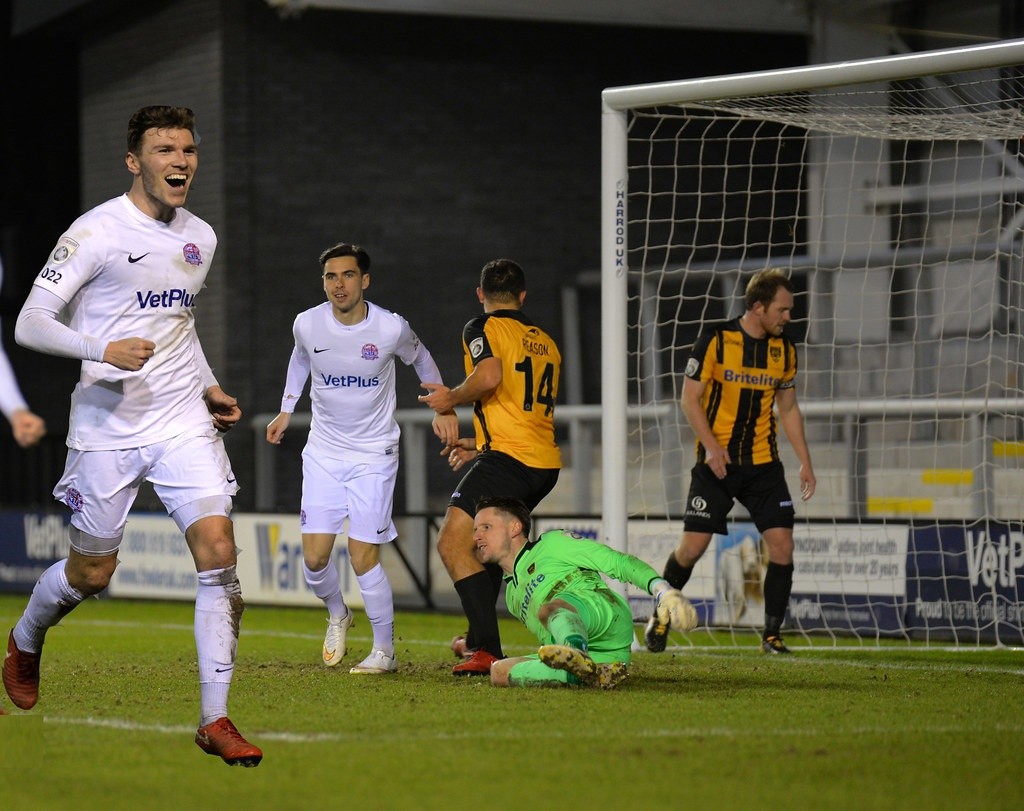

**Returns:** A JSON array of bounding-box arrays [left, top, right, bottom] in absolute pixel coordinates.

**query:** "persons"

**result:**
[[469, 492, 700, 692], [645, 265, 817, 658], [265, 241, 459, 676], [0, 346, 47, 448], [418, 259, 563, 676], [2, 105, 262, 770]]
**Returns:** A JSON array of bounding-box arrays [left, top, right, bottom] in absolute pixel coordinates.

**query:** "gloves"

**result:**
[[656, 589, 698, 632]]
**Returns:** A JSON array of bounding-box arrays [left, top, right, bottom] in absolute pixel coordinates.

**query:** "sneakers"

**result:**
[[538, 643, 596, 678], [582, 661, 635, 691], [759, 636, 792, 655], [451, 649, 500, 676], [321, 606, 354, 668], [450, 635, 475, 659], [2, 627, 41, 710], [195, 718, 262, 769], [643, 604, 671, 653], [347, 651, 398, 677]]
